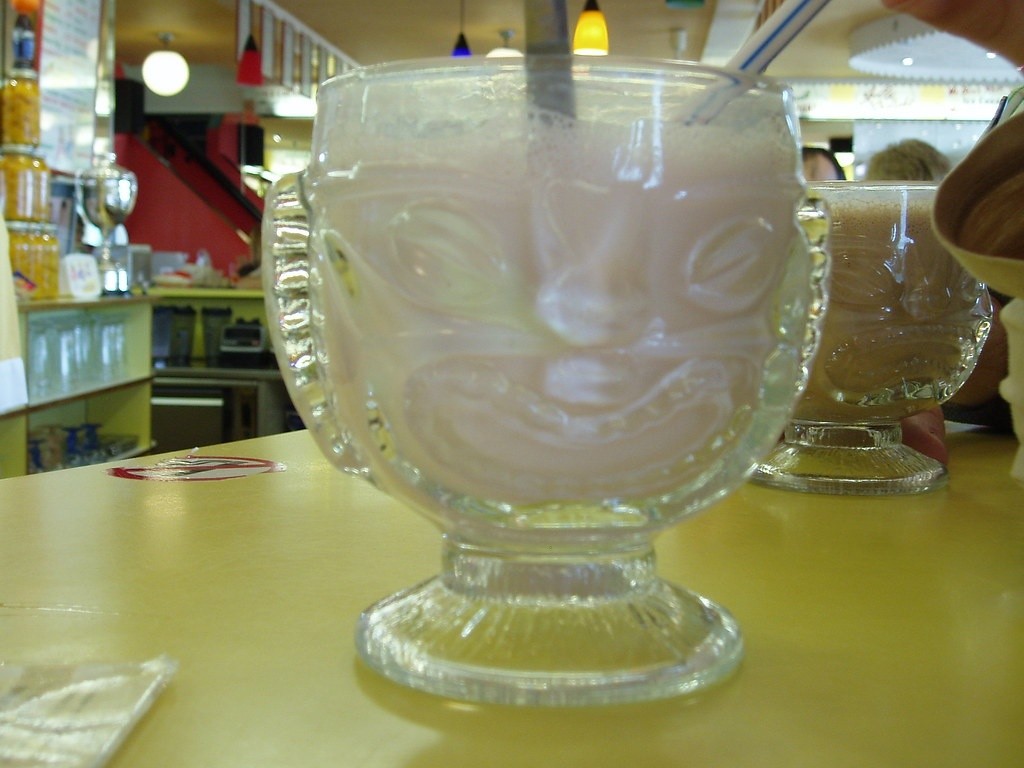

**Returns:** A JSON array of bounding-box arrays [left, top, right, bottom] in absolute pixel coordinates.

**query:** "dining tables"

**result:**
[[0, 421, 1024, 768]]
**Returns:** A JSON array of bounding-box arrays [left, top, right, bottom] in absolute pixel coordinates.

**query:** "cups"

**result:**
[[1, 65, 61, 304], [262, 168, 374, 487]]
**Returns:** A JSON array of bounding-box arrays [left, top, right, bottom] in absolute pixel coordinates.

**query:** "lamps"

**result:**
[[450, 0, 472, 57], [237, 1, 264, 86], [573, 0, 609, 56], [141, 31, 189, 97]]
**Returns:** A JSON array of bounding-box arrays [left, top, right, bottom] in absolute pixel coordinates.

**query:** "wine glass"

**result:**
[[748, 180, 994, 499], [306, 58, 805, 712]]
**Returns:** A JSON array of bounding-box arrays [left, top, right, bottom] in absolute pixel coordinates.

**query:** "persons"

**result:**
[[865, 138, 1016, 466], [880, 0, 1024, 488], [802, 147, 846, 181]]
